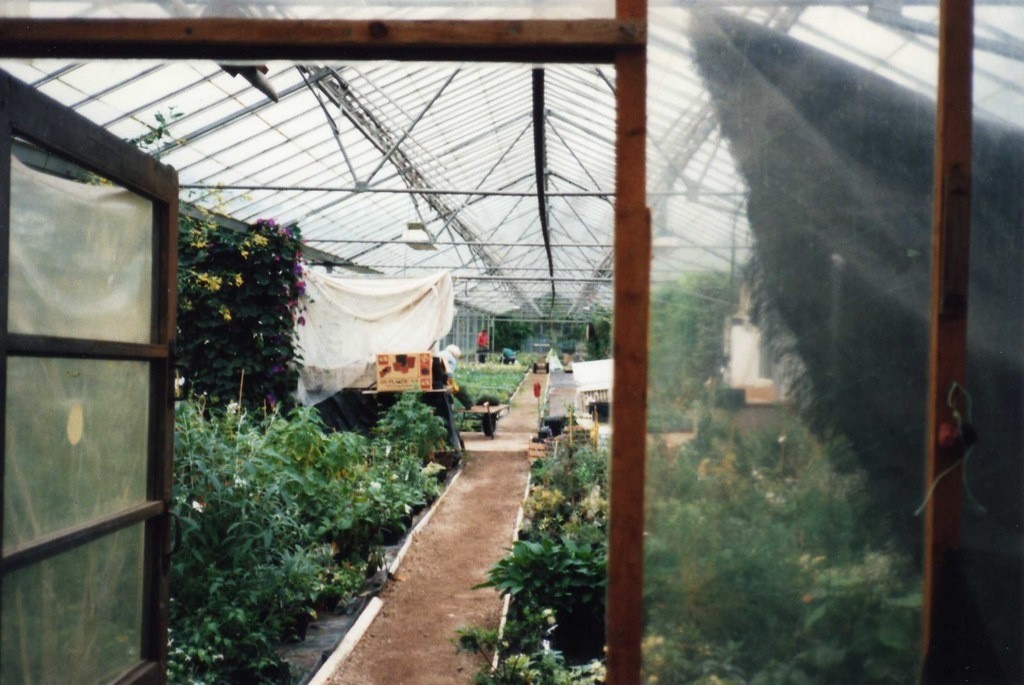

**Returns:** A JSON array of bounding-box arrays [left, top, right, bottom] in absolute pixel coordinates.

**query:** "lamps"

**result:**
[[401, 222, 435, 244]]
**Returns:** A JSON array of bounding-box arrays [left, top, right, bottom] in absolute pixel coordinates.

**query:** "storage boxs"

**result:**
[[376, 353, 433, 392]]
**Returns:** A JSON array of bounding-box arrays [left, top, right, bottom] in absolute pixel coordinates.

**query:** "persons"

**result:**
[[500, 343, 517, 365], [477, 329, 489, 363], [436, 344, 479, 411]]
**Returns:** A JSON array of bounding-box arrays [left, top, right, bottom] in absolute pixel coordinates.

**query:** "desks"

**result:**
[[322, 385, 465, 459], [457, 402, 511, 439]]
